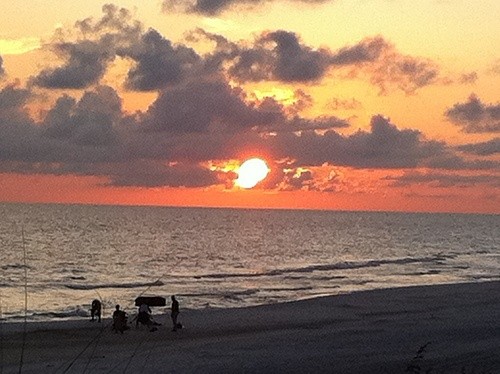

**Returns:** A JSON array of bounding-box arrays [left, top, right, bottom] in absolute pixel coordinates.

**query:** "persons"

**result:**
[[112, 305, 125, 334], [138, 304, 152, 329], [89, 299, 101, 322], [171, 295, 183, 331]]
